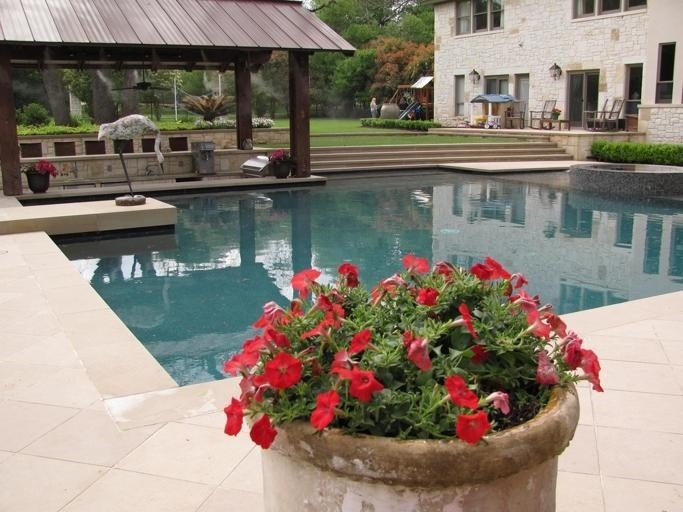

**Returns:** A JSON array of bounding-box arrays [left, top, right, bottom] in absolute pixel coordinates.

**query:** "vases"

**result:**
[[26, 171, 49, 194], [245, 383, 581, 512], [272, 160, 290, 178]]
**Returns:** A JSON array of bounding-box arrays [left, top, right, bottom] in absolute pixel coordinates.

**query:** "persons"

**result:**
[[369, 98, 378, 119]]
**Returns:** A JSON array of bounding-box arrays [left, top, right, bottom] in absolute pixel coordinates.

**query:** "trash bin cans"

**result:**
[[192, 142, 216, 175]]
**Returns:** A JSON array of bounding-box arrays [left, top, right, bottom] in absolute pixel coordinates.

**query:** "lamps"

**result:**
[[468, 69, 480, 85], [549, 63, 562, 80]]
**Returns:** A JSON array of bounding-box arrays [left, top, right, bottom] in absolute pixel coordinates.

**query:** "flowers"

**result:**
[[269, 149, 289, 160], [224, 255, 605, 449], [21, 160, 59, 177]]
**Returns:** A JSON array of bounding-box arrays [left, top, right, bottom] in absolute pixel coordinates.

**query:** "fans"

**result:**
[[111, 58, 170, 91]]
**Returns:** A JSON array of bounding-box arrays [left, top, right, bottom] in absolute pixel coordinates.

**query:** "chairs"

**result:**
[[505, 98, 624, 130]]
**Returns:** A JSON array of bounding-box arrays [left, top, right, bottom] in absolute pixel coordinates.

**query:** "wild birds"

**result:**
[[98, 114, 165, 197]]
